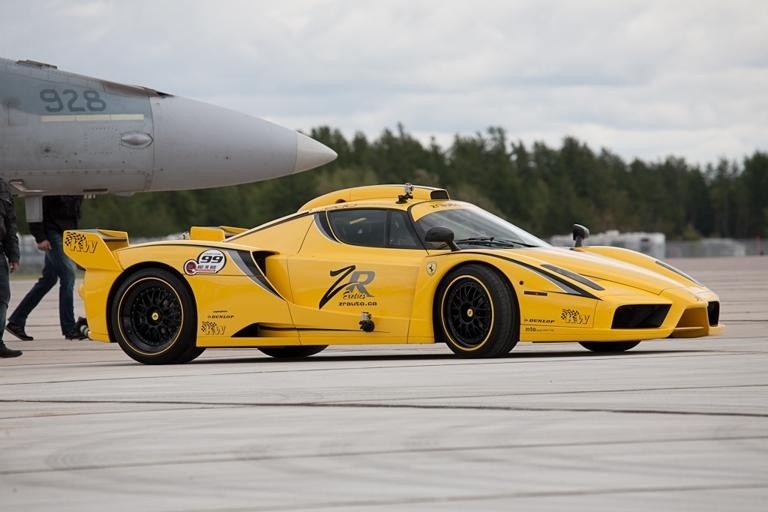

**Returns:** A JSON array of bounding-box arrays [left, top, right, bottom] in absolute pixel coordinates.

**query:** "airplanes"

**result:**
[[0, 57, 338, 223]]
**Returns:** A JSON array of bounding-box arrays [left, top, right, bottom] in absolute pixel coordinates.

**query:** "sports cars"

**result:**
[[63, 184, 720, 362]]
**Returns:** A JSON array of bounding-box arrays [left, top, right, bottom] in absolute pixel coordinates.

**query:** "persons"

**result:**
[[0, 176, 22, 358], [6, 195, 87, 341]]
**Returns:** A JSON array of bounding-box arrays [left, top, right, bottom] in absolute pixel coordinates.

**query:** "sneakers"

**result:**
[[6, 322, 33, 340], [66, 331, 85, 339], [0, 344, 23, 357]]
